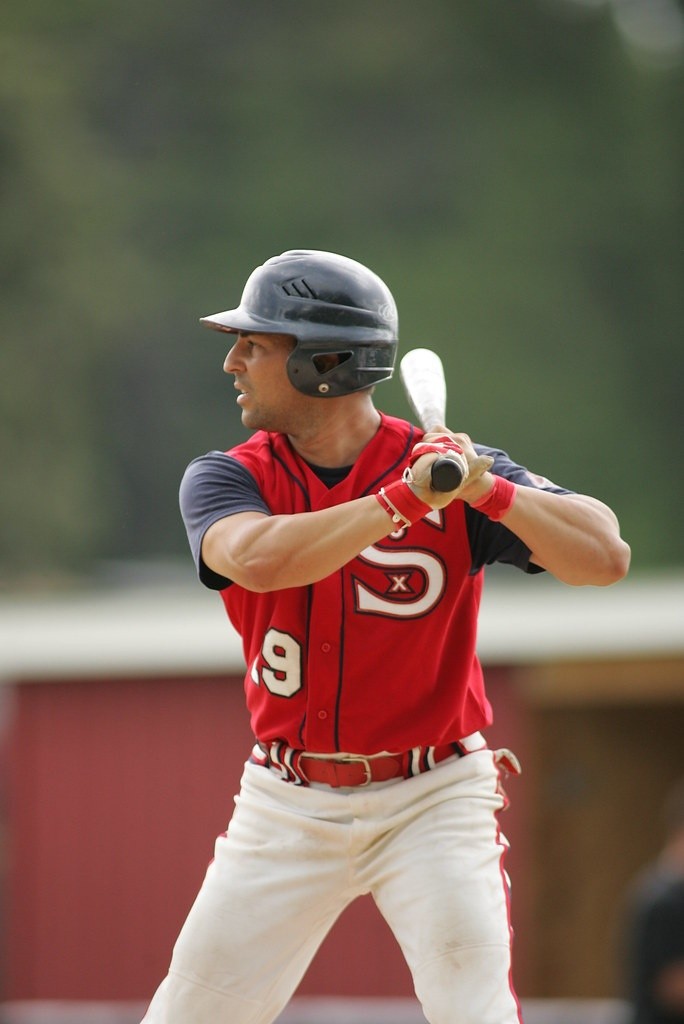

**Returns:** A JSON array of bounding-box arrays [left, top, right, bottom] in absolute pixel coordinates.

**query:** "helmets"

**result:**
[[199, 250, 400, 397]]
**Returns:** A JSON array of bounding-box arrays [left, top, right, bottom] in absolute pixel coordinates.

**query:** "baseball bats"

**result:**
[[396, 344, 462, 493]]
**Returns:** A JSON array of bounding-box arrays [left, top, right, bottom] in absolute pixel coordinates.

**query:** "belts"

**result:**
[[299, 742, 457, 787]]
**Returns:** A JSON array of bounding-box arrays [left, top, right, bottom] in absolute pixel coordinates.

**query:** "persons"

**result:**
[[139, 247, 638, 1024]]
